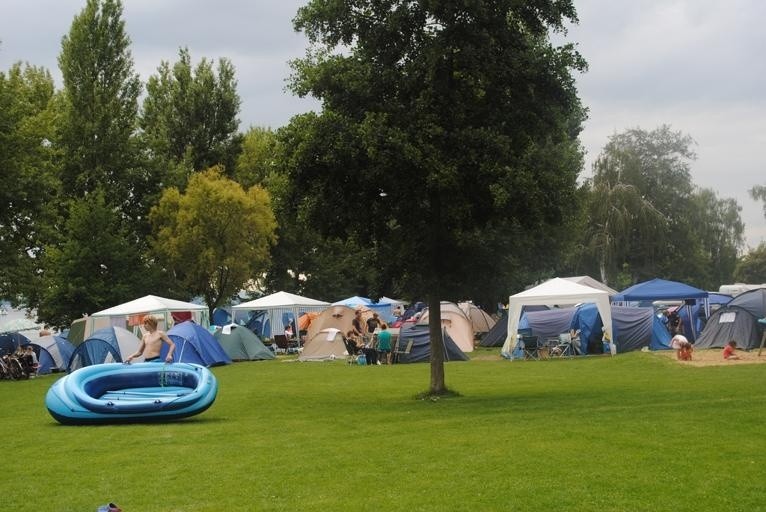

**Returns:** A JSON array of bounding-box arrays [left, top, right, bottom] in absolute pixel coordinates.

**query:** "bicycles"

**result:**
[[0, 347, 33, 382]]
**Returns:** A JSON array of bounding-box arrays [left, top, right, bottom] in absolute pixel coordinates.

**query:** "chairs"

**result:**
[[274, 335, 294, 355], [393, 338, 414, 364], [518, 328, 574, 361], [341, 335, 366, 367]]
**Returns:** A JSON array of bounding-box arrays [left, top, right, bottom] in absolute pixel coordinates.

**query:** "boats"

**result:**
[[45, 359, 218, 425]]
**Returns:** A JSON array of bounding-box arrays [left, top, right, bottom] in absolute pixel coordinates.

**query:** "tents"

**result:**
[[296, 301, 382, 363], [209, 320, 276, 363], [67, 325, 146, 373], [19, 334, 76, 375], [390, 275, 766, 366], [0, 317, 42, 359], [161, 319, 234, 372]]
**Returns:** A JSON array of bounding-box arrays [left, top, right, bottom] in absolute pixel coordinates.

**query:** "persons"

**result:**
[[22, 345, 40, 379], [12, 343, 25, 358], [284, 324, 293, 354], [39, 324, 51, 338], [347, 309, 391, 365], [120, 314, 175, 365]]
